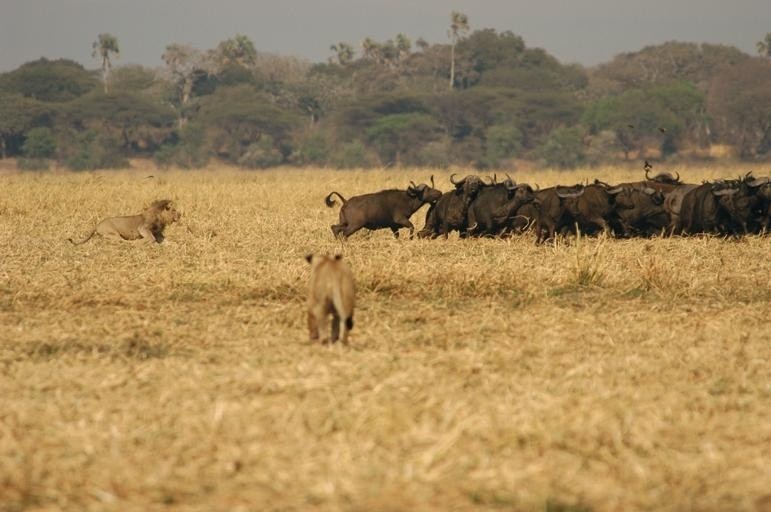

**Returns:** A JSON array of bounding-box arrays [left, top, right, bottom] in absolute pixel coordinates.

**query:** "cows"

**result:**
[[323, 168, 771, 247]]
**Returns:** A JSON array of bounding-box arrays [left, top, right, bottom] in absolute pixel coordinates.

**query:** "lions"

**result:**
[[303, 251, 357, 346], [66, 198, 182, 246]]
